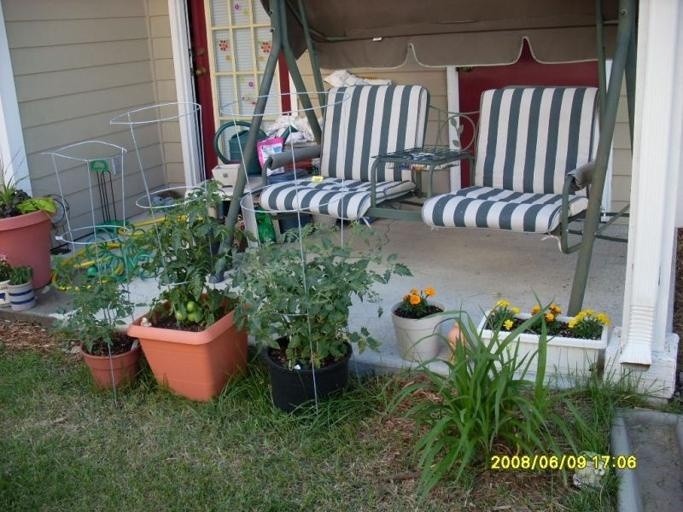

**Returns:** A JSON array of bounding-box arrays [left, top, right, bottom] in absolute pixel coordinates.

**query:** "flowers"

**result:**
[[399, 286, 436, 309], [492, 300, 610, 334]]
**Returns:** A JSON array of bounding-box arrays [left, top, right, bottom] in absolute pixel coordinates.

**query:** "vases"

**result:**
[[390, 303, 445, 360], [478, 309, 607, 378]]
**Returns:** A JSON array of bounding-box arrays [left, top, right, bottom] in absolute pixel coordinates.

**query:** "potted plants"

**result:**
[[233, 219, 409, 411], [0, 261, 11, 304], [53, 241, 139, 388], [122, 182, 255, 402], [0, 170, 55, 291], [7, 267, 38, 311]]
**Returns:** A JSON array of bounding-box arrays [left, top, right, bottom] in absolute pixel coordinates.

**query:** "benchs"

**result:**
[[209, 0, 640, 320]]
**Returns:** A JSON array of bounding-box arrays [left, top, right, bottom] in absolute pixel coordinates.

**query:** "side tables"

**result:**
[[196, 175, 266, 212]]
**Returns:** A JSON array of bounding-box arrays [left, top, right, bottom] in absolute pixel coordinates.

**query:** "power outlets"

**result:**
[[112, 156, 128, 174]]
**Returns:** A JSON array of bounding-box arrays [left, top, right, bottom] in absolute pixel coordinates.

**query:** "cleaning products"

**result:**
[[257, 138, 284, 175]]
